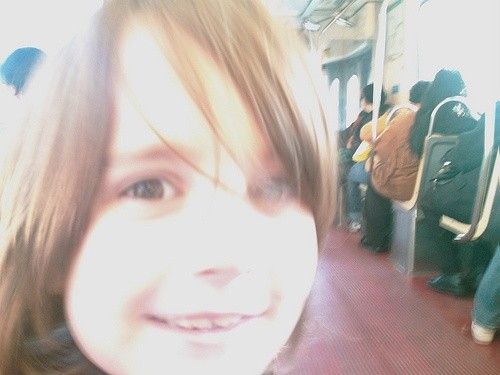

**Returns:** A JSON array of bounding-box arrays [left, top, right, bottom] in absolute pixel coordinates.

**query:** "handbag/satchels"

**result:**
[[351, 141, 373, 163]]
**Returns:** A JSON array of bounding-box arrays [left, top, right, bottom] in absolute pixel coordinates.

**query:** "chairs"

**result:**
[[359, 96, 500, 275]]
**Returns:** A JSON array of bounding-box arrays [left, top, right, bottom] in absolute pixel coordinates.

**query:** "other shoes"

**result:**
[[471, 319, 496, 346], [348, 220, 362, 233], [360, 235, 387, 253], [428, 271, 471, 296]]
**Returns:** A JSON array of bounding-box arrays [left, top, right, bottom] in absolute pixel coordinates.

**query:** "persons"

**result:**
[[0, 47, 59, 98], [337, 69, 500, 344], [0, 0, 336, 375]]
